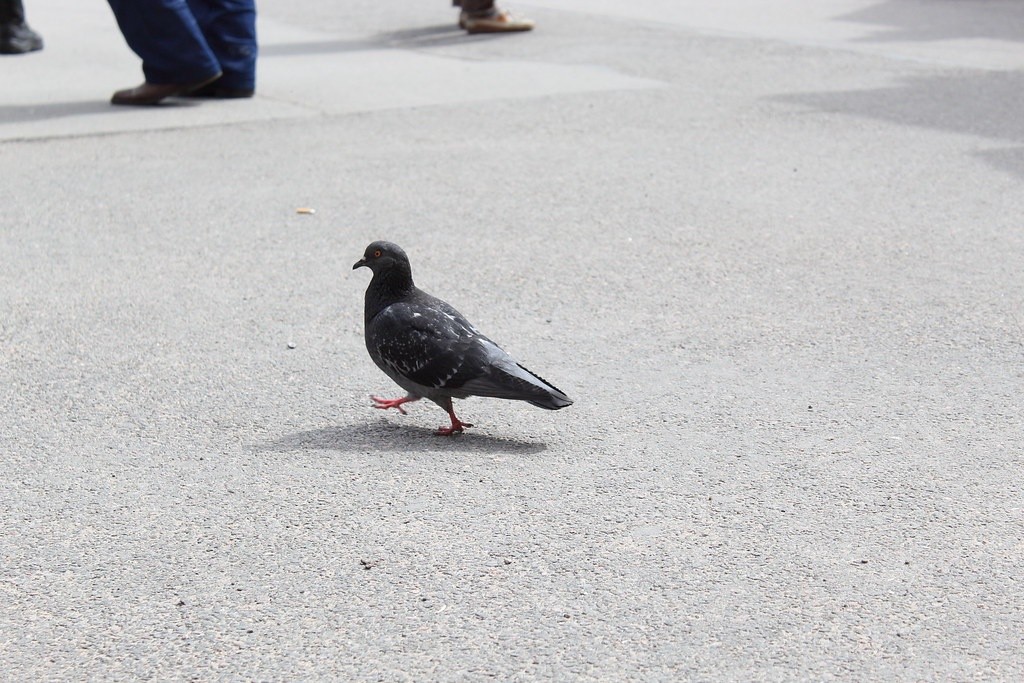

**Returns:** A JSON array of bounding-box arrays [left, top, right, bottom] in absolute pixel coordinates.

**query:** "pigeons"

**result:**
[[352, 239, 576, 438]]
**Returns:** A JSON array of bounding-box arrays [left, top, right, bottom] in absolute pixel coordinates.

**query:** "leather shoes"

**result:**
[[111, 71, 254, 105]]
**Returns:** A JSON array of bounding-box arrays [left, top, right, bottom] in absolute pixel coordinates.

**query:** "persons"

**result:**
[[451, 0, 535, 34], [0, 0, 43, 53], [106, 1, 258, 103]]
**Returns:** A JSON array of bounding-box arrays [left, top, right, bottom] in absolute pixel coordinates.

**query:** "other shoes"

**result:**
[[459, 11, 534, 33]]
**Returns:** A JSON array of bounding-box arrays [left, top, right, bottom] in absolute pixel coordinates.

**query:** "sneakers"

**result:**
[[0, 22, 42, 54]]
